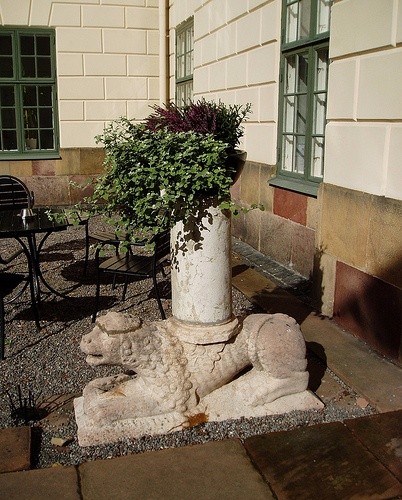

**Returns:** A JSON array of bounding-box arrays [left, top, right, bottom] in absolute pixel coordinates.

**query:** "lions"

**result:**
[[80, 311, 310, 423]]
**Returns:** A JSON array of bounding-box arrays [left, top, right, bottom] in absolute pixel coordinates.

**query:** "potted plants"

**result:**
[[45, 98, 267, 264]]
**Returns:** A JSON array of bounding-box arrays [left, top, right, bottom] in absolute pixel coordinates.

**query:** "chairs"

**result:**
[[75, 198, 159, 269], [89, 224, 174, 326], [0, 172, 46, 273]]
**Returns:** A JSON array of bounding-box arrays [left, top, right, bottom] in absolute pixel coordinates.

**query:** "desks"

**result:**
[[0, 205, 94, 337]]
